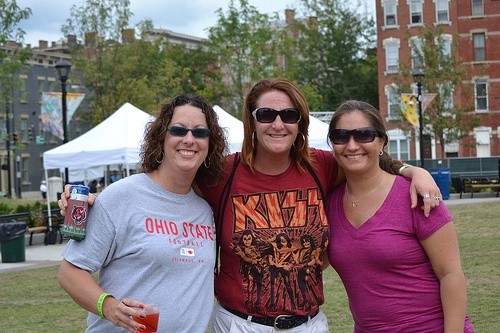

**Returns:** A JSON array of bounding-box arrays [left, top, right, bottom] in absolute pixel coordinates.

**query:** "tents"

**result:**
[[308, 115, 333, 150], [42, 102, 157, 233], [212, 104, 244, 156]]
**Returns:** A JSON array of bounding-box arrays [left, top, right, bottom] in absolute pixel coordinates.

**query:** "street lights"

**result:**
[[411, 69, 427, 168], [16, 155, 22, 199], [54, 58, 73, 184]]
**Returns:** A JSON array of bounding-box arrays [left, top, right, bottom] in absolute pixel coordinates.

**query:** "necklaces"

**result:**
[[345, 170, 384, 207]]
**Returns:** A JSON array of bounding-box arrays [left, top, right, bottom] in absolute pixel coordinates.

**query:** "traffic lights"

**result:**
[[27, 127, 33, 141], [36, 136, 45, 145], [13, 133, 19, 142]]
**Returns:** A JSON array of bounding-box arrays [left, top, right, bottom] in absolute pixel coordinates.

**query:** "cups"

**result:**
[[130, 305, 160, 333]]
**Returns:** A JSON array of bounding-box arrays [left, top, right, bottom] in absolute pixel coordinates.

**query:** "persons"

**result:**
[[90, 180, 97, 193], [57, 96, 228, 333], [99, 176, 113, 186], [321, 100, 475, 333], [57, 78, 443, 333]]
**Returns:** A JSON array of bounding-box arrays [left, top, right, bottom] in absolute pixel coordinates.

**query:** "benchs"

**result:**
[[460, 176, 500, 199]]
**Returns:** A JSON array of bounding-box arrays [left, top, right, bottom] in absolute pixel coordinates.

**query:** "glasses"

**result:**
[[328, 126, 381, 145], [251, 107, 302, 124], [165, 125, 210, 139]]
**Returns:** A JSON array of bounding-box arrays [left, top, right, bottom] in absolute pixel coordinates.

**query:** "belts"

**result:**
[[219, 302, 319, 329]]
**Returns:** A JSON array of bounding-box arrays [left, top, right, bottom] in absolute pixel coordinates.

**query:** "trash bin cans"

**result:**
[[0, 222, 28, 263]]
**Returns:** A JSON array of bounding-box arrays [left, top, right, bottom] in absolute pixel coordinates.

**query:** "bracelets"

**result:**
[[97, 293, 116, 319], [399, 165, 411, 175]]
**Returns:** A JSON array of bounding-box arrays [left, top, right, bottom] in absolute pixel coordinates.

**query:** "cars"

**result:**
[[40, 177, 64, 199]]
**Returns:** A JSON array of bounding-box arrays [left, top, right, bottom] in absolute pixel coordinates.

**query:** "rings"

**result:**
[[423, 195, 430, 198], [435, 198, 440, 200]]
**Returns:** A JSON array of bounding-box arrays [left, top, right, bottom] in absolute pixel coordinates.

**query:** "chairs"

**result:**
[[28, 210, 63, 245]]
